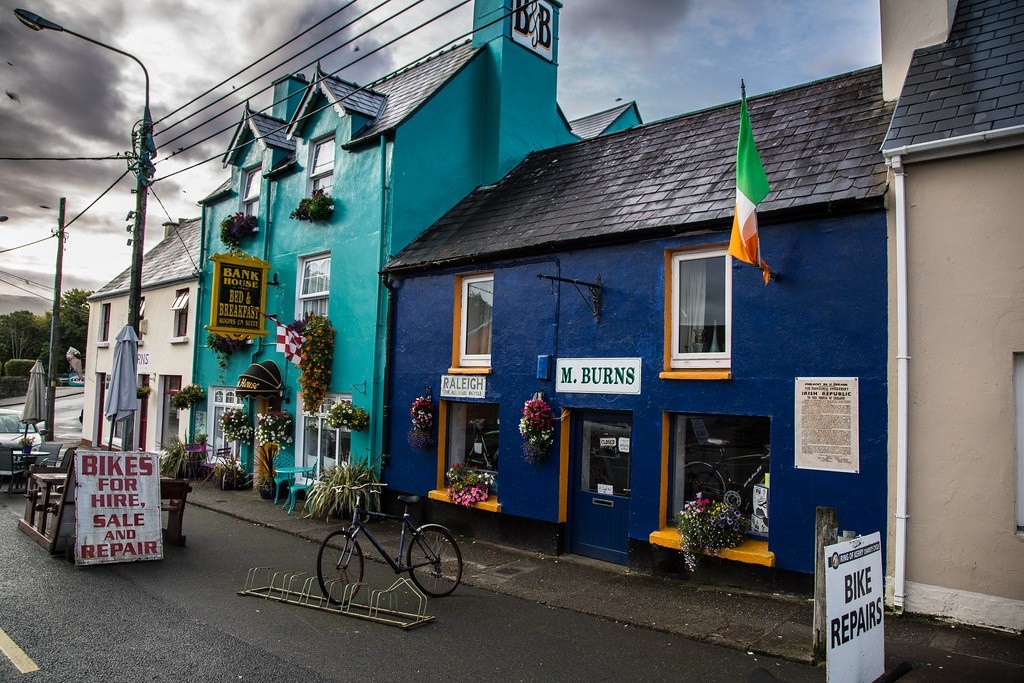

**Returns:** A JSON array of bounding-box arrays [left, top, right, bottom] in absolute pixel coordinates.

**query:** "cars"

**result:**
[[0, 410, 49, 478]]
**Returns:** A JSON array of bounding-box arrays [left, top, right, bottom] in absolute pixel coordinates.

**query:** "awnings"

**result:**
[[234, 359, 287, 394]]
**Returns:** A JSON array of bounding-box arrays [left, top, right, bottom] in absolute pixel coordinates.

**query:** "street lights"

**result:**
[[13, 7, 149, 448]]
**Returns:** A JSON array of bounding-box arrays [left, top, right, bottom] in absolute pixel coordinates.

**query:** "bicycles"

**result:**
[[685, 437, 771, 515], [317, 483, 463, 604]]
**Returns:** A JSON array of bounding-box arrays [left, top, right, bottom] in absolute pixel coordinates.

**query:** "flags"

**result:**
[[256, 310, 304, 367], [726, 81, 770, 286]]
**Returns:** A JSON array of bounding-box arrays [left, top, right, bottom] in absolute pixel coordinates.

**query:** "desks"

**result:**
[[12, 451, 50, 494], [274, 467, 314, 508], [186, 449, 215, 481]]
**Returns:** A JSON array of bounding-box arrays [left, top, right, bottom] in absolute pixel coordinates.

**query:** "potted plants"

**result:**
[[256, 441, 280, 499], [214, 457, 246, 490], [206, 334, 229, 385], [304, 193, 334, 222], [136, 384, 150, 399], [18, 436, 35, 454], [219, 215, 240, 256], [172, 385, 205, 410]]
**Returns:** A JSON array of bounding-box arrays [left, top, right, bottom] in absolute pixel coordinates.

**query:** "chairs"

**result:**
[[183, 444, 205, 483], [272, 452, 297, 504], [0, 444, 78, 498], [282, 459, 319, 515], [199, 447, 231, 486]]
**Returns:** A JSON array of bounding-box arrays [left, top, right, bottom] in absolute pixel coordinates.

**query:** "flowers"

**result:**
[[231, 212, 258, 237], [517, 391, 555, 464], [673, 493, 751, 573], [218, 408, 293, 450], [289, 189, 324, 222], [213, 334, 250, 351], [408, 392, 435, 453], [444, 463, 496, 508], [289, 310, 334, 431], [327, 399, 369, 433]]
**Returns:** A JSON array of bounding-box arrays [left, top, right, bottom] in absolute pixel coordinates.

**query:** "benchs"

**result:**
[[18, 464, 192, 555]]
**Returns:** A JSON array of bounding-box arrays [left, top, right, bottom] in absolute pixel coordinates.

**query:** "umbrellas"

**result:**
[[19, 358, 48, 438], [105, 324, 140, 451]]
